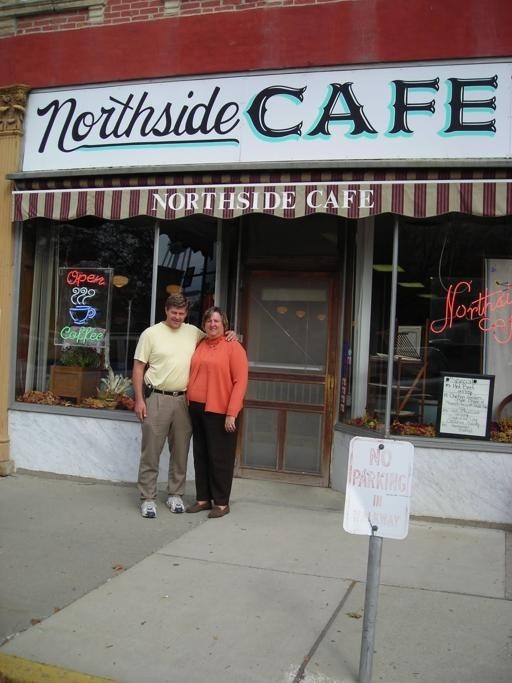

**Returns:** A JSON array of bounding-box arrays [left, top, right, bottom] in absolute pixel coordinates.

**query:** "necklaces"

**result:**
[[206, 337, 222, 348]]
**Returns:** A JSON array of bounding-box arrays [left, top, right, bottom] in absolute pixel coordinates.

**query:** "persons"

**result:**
[[131, 289, 241, 519], [185, 304, 249, 520]]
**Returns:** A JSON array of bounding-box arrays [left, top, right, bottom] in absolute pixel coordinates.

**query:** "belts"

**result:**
[[153, 388, 186, 397]]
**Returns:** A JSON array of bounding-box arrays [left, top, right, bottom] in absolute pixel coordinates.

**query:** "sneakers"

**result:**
[[186, 502, 212, 513], [209, 505, 231, 519], [167, 496, 186, 513], [140, 499, 157, 518]]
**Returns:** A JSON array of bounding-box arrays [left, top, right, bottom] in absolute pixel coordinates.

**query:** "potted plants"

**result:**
[[48, 346, 104, 404]]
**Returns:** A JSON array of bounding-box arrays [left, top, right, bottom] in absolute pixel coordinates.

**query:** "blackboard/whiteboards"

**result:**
[[55, 267, 111, 348]]
